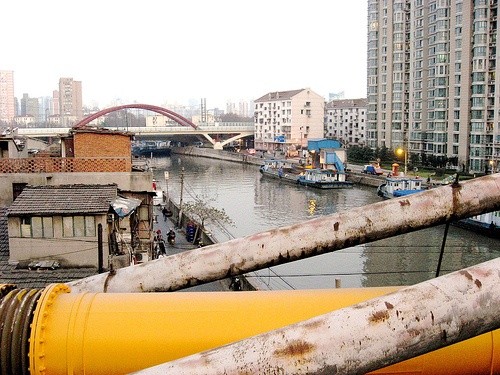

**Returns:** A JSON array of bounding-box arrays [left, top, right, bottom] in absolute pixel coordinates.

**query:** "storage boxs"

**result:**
[[185, 225, 195, 242]]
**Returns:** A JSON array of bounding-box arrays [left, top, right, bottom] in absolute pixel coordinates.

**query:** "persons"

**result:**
[[166, 228, 176, 242], [162, 207, 170, 222], [153, 180, 156, 192], [229, 278, 242, 291]]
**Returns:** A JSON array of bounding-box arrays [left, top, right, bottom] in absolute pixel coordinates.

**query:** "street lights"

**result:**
[[490, 161, 494, 174], [397, 149, 406, 175]]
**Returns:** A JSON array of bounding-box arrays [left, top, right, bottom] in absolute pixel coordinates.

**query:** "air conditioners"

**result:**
[[135, 252, 148, 263]]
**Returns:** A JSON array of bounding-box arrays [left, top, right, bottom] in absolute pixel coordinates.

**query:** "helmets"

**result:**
[[169, 228, 173, 231]]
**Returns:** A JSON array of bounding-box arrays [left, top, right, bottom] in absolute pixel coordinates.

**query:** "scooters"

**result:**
[[186, 225, 193, 241], [167, 231, 175, 247]]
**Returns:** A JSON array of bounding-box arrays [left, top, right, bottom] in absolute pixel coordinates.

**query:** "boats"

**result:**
[[132, 156, 149, 172], [258, 159, 354, 189], [376, 177, 500, 238]]
